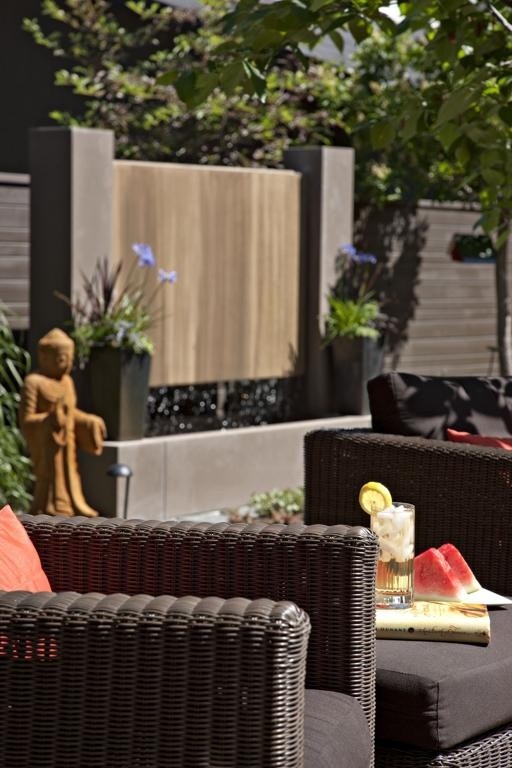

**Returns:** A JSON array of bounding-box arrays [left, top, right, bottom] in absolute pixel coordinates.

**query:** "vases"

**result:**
[[324, 338, 384, 415], [84, 350, 153, 440]]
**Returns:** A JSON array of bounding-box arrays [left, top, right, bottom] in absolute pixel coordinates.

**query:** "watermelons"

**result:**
[[437, 543, 482, 593], [413, 547, 470, 604]]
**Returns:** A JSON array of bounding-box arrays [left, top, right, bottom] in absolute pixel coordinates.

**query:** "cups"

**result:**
[[371, 502, 415, 610]]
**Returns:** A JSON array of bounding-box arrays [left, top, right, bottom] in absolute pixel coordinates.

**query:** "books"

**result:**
[[377, 599, 491, 647]]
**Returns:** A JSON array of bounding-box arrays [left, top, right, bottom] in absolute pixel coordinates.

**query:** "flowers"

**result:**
[[317, 244, 395, 337], [53, 242, 177, 350]]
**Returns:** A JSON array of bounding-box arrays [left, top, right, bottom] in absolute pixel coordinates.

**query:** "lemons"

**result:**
[[359, 481, 392, 516]]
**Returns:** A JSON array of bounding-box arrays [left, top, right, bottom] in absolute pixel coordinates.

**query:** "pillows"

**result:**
[[367, 372, 511, 444], [1, 504, 56, 659], [447, 428, 512, 450]]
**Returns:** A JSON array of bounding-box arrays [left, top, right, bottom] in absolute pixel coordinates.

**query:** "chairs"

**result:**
[[1, 513, 379, 768], [304, 372, 512, 597]]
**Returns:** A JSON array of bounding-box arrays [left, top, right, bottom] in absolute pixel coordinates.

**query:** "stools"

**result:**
[[376, 598, 511, 768]]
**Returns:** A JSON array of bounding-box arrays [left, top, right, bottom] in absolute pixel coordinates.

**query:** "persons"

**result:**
[[17, 326, 109, 519]]
